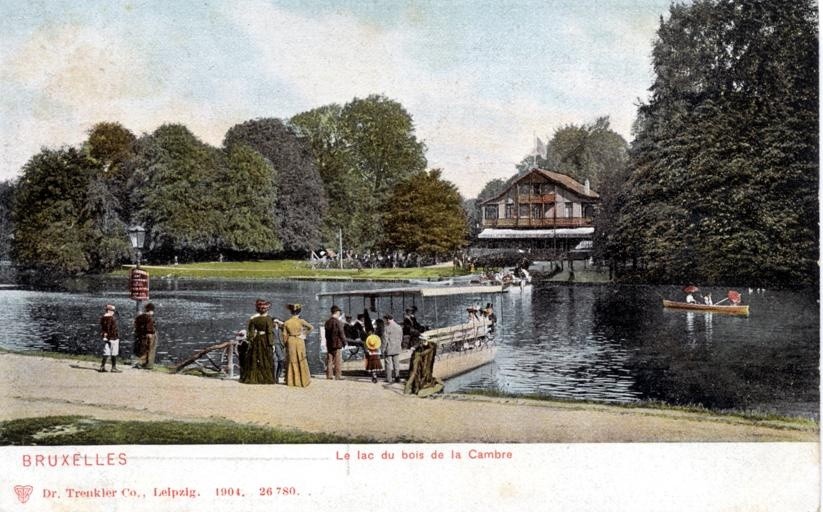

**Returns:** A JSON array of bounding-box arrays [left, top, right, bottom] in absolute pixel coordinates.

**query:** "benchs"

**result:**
[[410, 320, 497, 361], [319, 321, 410, 366]]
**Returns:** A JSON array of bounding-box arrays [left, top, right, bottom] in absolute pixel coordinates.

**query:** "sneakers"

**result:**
[[371, 377, 400, 384]]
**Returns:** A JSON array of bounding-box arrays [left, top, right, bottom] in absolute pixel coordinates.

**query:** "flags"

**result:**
[[535, 137, 547, 160]]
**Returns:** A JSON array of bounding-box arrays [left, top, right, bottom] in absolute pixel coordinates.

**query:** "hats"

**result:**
[[365, 333, 381, 349], [255, 297, 271, 311], [465, 305, 480, 312], [288, 302, 304, 312], [105, 304, 115, 310]]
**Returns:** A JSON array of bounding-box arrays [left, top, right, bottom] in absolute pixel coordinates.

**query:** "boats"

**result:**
[[316, 274, 513, 381], [663, 299, 750, 317]]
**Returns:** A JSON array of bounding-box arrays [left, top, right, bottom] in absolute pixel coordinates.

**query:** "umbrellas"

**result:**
[[681, 286, 700, 293], [728, 291, 741, 303]]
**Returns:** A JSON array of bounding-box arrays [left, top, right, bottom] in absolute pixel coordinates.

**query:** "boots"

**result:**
[[98, 355, 108, 372], [110, 355, 122, 373]]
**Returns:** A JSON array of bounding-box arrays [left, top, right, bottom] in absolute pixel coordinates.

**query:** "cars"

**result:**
[[473, 249, 528, 266], [570, 241, 594, 260]]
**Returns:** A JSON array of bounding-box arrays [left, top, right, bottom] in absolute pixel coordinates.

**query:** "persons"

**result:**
[[132, 302, 158, 368], [230, 297, 496, 387], [97, 303, 124, 373], [685, 291, 698, 303], [704, 292, 714, 306], [338, 241, 478, 273]]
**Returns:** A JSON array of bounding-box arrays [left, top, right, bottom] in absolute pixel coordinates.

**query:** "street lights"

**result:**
[[127, 221, 150, 365]]
